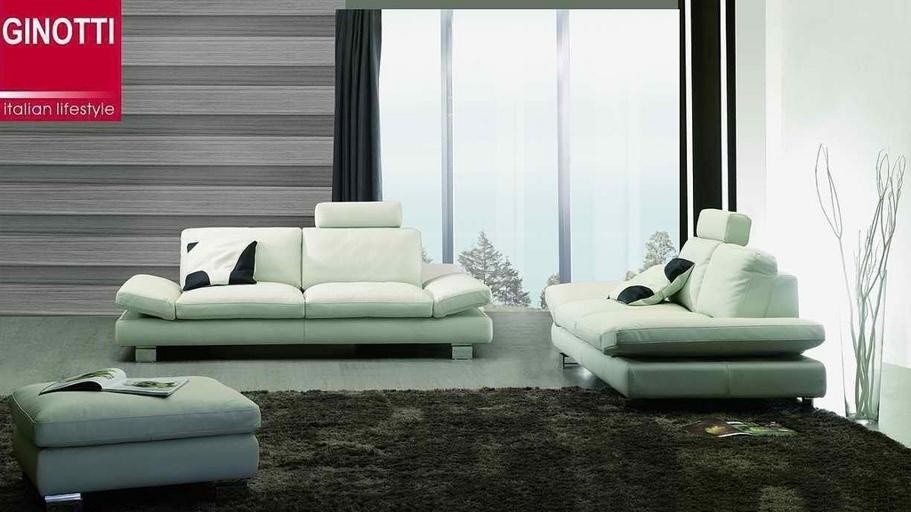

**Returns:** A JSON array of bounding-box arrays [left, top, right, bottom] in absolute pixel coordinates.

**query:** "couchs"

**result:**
[[112, 200, 493, 363], [545, 208, 827, 405]]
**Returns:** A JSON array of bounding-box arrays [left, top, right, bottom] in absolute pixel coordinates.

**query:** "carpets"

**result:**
[[0, 386, 910, 508]]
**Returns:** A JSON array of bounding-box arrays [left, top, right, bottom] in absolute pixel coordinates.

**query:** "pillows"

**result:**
[[183, 240, 258, 291], [606, 257, 694, 306]]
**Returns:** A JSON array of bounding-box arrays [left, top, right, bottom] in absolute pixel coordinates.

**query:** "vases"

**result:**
[[838, 270, 888, 425]]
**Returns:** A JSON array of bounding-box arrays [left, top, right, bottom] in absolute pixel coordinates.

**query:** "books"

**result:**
[[38, 365, 189, 396], [679, 416, 798, 439]]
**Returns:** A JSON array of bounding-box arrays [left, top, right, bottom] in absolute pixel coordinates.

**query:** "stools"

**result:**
[[7, 376, 263, 504]]
